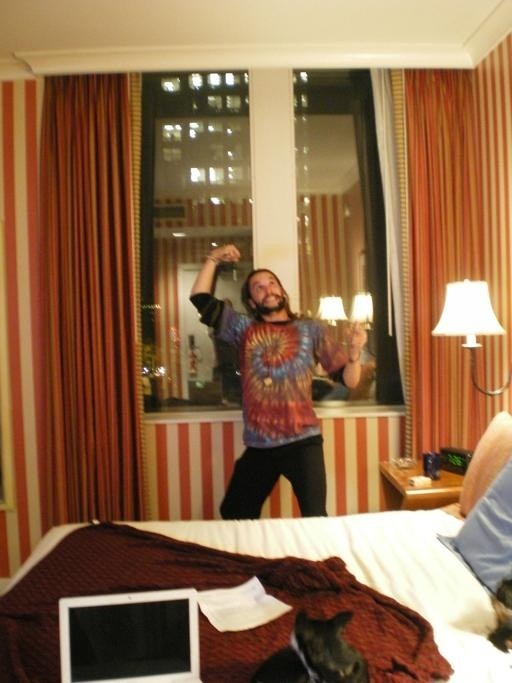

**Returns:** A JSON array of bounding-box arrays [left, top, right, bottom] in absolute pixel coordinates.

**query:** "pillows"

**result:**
[[434, 452, 511, 596], [456, 410, 512, 518]]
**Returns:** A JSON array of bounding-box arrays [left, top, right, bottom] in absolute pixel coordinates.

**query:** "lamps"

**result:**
[[314, 295, 349, 330], [346, 293, 373, 334], [428, 277, 512, 400]]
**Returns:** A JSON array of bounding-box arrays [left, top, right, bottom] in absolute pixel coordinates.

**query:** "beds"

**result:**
[[0, 497, 510, 683]]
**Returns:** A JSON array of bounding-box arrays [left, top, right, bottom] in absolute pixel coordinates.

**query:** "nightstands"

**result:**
[[375, 458, 467, 512]]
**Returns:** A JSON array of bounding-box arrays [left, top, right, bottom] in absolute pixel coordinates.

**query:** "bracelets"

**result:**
[[347, 351, 361, 363], [205, 253, 223, 266]]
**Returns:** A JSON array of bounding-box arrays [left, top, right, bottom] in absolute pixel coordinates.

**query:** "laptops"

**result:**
[[58, 587, 203, 683]]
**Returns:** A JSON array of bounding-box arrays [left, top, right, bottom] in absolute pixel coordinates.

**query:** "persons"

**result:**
[[189, 243, 369, 520]]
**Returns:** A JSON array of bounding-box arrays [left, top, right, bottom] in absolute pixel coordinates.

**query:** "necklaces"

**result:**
[[261, 315, 291, 387]]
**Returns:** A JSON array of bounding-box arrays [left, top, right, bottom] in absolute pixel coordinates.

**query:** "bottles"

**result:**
[[142, 365, 156, 413], [155, 366, 168, 407], [185, 333, 199, 378]]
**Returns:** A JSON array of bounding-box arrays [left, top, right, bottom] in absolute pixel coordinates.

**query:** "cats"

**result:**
[[453, 579, 512, 653], [249, 609, 369, 683]]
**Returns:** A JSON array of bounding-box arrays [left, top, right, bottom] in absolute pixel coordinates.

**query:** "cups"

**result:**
[[424, 452, 441, 481]]
